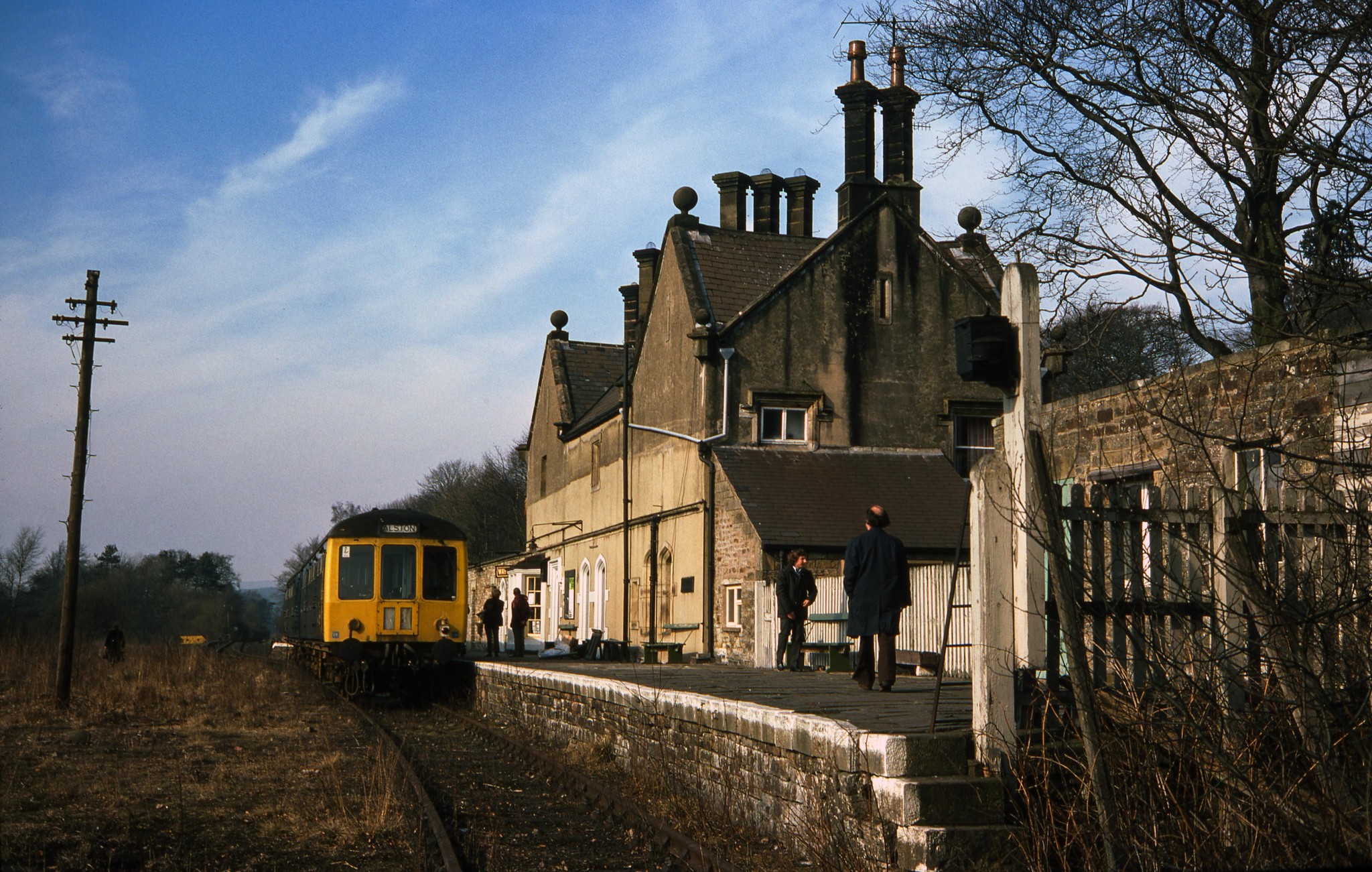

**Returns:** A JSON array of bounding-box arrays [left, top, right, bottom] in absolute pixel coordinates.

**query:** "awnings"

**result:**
[[505, 552, 545, 576]]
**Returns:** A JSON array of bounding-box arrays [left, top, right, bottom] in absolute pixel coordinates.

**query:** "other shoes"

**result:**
[[790, 666, 804, 673], [484, 653, 492, 656], [857, 681, 873, 690], [879, 681, 892, 692], [495, 653, 500, 657], [777, 660, 785, 670], [509, 653, 524, 657]]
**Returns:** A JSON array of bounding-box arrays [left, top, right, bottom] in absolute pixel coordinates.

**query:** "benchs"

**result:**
[[600, 641, 633, 660], [640, 642, 685, 663]]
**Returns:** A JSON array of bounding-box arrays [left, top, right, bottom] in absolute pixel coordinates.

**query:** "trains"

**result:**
[[282, 507, 470, 702]]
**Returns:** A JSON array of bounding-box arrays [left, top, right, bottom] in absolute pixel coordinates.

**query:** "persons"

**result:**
[[843, 505, 912, 692], [776, 548, 818, 671], [509, 587, 530, 657], [482, 589, 504, 657], [105, 624, 125, 668]]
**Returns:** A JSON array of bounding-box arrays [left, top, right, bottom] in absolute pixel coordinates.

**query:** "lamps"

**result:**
[[529, 520, 583, 553]]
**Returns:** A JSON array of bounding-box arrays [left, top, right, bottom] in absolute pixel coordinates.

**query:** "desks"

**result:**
[[783, 642, 855, 672]]
